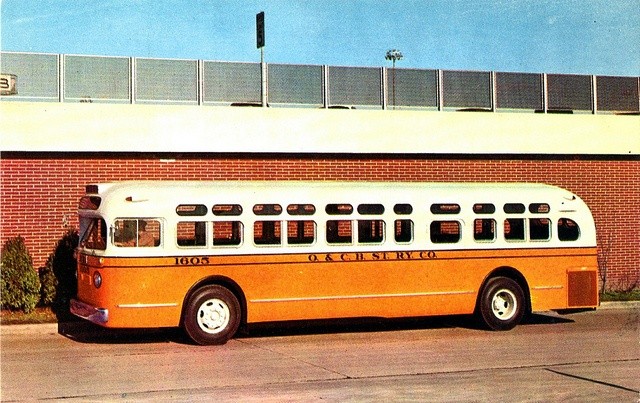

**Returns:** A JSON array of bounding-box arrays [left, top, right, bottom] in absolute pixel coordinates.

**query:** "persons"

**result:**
[[114, 219, 155, 247]]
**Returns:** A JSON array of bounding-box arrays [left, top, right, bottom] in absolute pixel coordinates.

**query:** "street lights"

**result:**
[[385, 48, 403, 110]]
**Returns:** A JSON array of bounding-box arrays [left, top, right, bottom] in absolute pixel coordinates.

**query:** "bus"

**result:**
[[69, 180, 600, 346]]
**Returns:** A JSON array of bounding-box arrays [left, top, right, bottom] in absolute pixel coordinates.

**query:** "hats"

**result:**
[[135, 220, 147, 230]]
